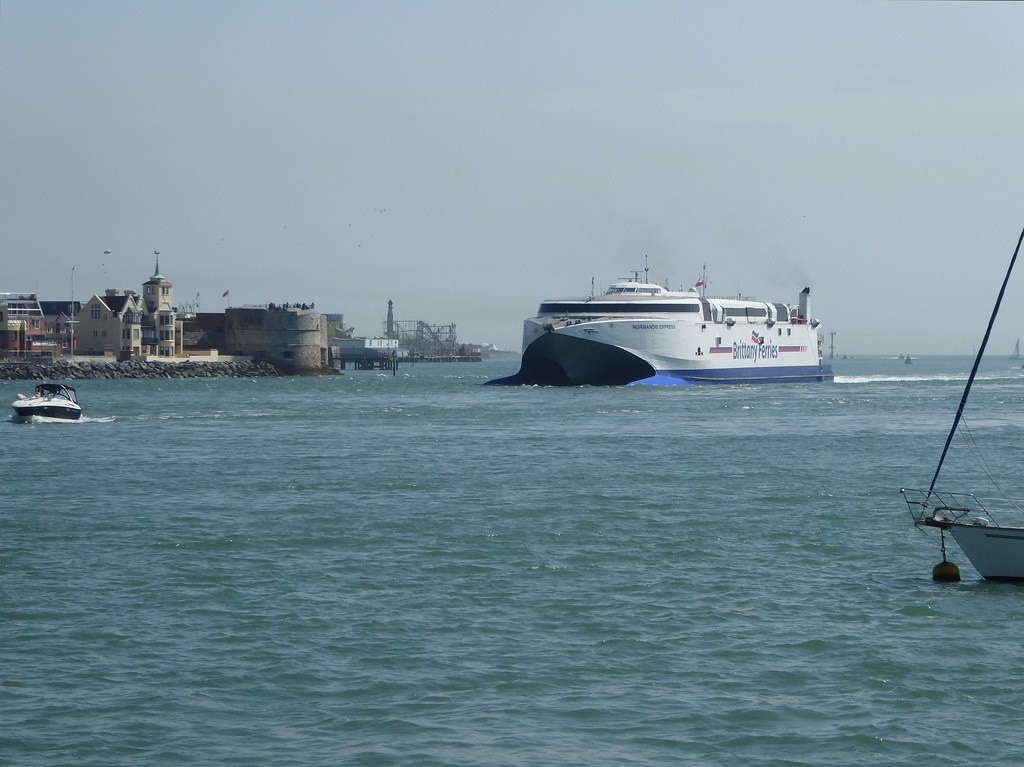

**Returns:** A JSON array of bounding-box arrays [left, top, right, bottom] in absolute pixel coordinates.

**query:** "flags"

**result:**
[[222, 291, 228, 297], [696, 279, 703, 286]]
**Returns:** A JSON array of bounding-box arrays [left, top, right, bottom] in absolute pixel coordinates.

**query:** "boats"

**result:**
[[899, 354, 904, 359], [12, 384, 82, 421], [905, 354, 912, 364], [482, 255, 835, 387], [901, 227, 1024, 583]]
